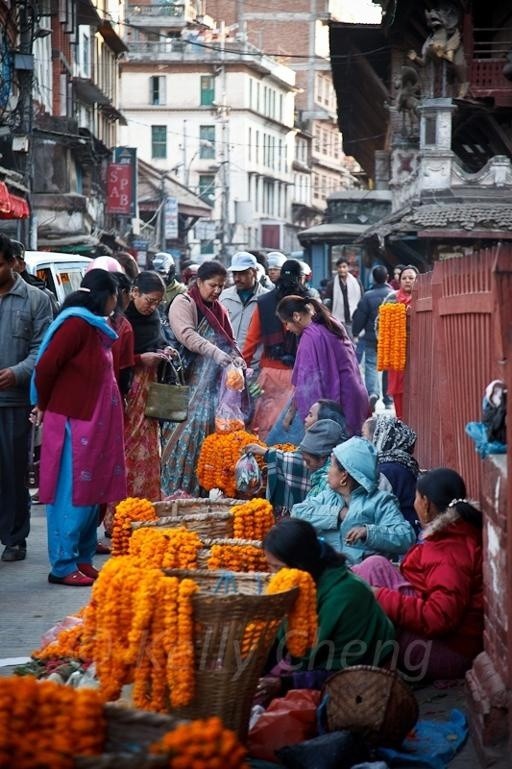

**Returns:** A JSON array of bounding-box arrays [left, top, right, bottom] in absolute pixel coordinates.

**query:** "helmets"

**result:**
[[87, 256, 123, 275]]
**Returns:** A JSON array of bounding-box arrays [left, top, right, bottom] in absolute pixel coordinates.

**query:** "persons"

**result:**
[[0, 233, 484, 705]]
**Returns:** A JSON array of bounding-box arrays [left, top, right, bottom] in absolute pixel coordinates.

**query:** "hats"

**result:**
[[300, 419, 342, 456], [267, 251, 287, 270], [228, 252, 257, 271]]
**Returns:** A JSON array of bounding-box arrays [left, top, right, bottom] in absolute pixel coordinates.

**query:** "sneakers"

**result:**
[[369, 394, 379, 412], [2, 542, 110, 586]]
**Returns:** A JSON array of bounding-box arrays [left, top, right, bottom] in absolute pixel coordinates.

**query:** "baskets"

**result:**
[[162, 569, 299, 743], [71, 702, 183, 769], [130, 498, 250, 539], [195, 538, 263, 571], [323, 665, 421, 749], [74, 498, 418, 768]]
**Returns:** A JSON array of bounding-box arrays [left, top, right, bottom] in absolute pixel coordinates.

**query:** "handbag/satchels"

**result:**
[[145, 382, 189, 423]]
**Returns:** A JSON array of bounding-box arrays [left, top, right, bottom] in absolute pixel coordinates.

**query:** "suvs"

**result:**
[[151, 252, 175, 274], [21, 250, 95, 307]]
